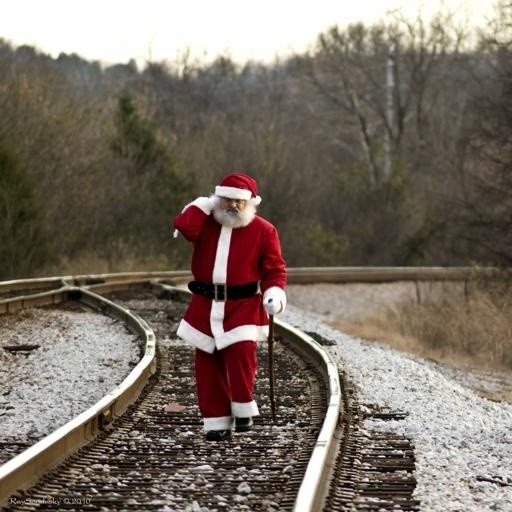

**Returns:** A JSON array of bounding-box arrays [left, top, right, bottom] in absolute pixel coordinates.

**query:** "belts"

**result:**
[[188, 280, 259, 301]]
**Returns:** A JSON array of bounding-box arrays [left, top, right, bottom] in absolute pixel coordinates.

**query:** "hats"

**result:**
[[214, 171, 263, 207]]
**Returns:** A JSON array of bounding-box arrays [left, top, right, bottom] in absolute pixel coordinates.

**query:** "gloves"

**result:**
[[264, 294, 281, 314]]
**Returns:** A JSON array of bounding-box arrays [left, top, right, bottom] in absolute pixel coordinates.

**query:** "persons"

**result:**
[[171, 171, 289, 442]]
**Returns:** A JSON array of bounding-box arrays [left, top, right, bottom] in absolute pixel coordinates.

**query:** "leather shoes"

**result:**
[[205, 412, 254, 442]]
